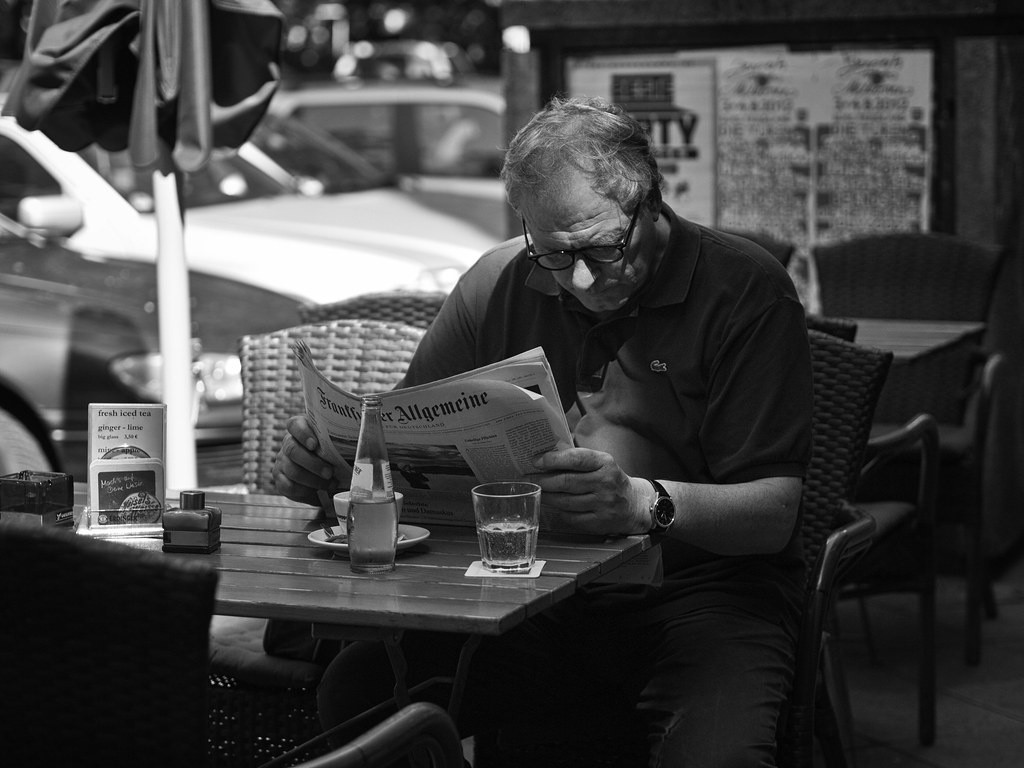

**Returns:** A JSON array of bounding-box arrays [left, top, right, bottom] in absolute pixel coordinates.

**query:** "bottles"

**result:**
[[347, 395, 398, 575], [162, 492, 221, 554]]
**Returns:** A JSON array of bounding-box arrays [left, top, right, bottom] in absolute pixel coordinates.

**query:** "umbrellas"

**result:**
[[0, 1, 284, 507]]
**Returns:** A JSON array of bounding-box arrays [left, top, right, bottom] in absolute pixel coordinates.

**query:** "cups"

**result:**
[[471, 482, 541, 574], [334, 490, 403, 542]]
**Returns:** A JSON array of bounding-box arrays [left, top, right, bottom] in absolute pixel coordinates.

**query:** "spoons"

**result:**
[[319, 521, 346, 542]]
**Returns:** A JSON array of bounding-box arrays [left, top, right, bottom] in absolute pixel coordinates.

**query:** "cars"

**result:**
[[0, 215, 319, 481], [0, 106, 508, 312], [265, 84, 515, 245]]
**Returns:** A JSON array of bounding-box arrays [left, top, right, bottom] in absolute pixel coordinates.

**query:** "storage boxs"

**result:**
[[0, 470, 74, 532]]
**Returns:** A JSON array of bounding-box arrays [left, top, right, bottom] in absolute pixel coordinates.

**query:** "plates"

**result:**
[[308, 525, 430, 556]]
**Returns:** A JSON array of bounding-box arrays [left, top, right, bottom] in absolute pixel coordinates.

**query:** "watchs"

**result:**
[[646, 478, 677, 540]]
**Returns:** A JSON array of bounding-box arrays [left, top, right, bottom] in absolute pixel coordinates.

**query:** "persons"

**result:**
[[272, 95, 807, 768]]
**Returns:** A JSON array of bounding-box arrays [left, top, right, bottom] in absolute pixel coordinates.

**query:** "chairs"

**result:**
[[720, 232, 796, 269], [803, 313, 946, 748], [812, 232, 1008, 669], [770, 331, 898, 768], [310, 294, 450, 331], [209, 317, 431, 688], [0, 511, 465, 768]]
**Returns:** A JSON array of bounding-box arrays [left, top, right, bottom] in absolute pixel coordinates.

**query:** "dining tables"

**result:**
[[64, 490, 668, 767], [819, 319, 989, 675]]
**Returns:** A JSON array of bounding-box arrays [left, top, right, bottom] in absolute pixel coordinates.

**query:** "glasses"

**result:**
[[521, 201, 641, 271]]
